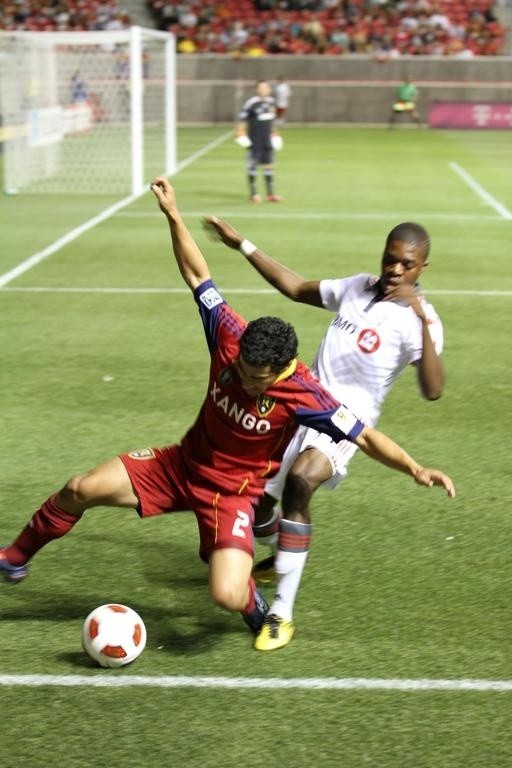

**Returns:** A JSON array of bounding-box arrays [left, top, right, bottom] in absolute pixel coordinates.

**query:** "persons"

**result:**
[[202, 213, 444, 650], [235, 80, 283, 202], [387, 74, 426, 130], [68, 65, 88, 101], [1, 178, 455, 636], [273, 75, 293, 117], [0, 1, 502, 57]]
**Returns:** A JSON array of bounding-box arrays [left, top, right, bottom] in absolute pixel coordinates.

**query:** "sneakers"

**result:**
[[244, 556, 294, 652], [250, 195, 279, 203], [0, 547, 28, 581]]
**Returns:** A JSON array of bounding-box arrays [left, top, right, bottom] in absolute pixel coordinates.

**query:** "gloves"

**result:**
[[271, 133, 282, 149], [235, 131, 252, 148]]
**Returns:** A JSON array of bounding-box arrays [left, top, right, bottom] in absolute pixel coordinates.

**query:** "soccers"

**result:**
[[79, 603, 150, 669]]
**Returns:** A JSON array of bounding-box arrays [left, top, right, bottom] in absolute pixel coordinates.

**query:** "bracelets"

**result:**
[[237, 238, 257, 257]]
